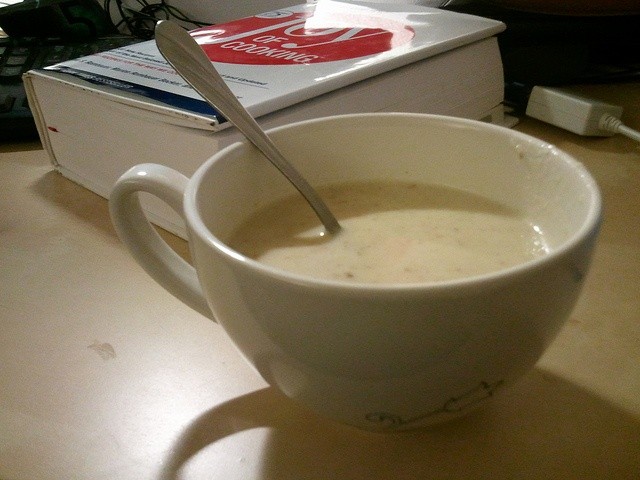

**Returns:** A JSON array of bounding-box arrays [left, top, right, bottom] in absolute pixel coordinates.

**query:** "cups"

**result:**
[[109, 112, 602, 434]]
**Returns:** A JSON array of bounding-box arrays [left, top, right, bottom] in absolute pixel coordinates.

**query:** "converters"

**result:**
[[525, 81, 625, 137]]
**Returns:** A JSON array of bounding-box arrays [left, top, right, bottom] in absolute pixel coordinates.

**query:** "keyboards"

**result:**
[[0, 34, 147, 138]]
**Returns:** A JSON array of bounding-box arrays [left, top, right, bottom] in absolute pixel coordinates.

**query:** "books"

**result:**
[[21, 0, 520, 248]]
